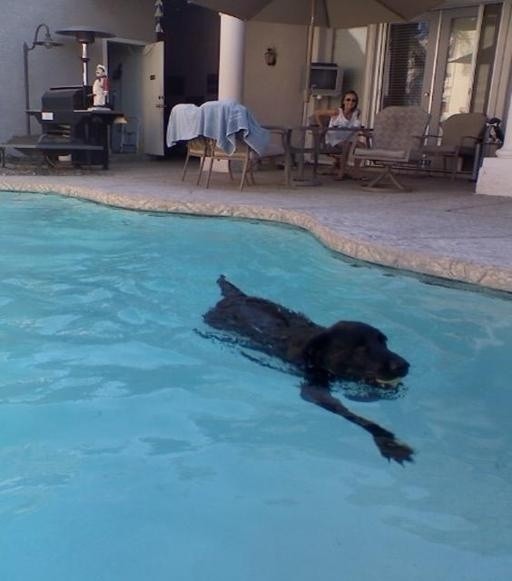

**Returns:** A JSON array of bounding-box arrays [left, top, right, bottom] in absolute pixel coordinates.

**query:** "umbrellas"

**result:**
[[188, 0, 443, 179]]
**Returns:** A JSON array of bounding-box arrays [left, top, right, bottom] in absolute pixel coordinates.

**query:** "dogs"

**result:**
[[193, 273, 417, 470]]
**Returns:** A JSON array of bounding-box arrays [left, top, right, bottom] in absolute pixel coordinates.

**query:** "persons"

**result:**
[[315, 91, 366, 181]]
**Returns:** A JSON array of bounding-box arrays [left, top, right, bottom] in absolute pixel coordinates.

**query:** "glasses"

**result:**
[[346, 98, 357, 102]]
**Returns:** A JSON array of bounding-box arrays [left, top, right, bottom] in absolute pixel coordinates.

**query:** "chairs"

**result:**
[[182, 136, 234, 185], [206, 126, 290, 192], [309, 106, 489, 193]]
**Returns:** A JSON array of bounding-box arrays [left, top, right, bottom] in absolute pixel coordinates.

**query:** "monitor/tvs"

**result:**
[[306, 65, 343, 96]]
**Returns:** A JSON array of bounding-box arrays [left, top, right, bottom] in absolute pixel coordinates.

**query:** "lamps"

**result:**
[[22, 25, 64, 136], [264, 47, 276, 65]]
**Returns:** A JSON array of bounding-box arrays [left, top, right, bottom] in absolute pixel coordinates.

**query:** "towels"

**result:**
[[165, 101, 269, 158]]
